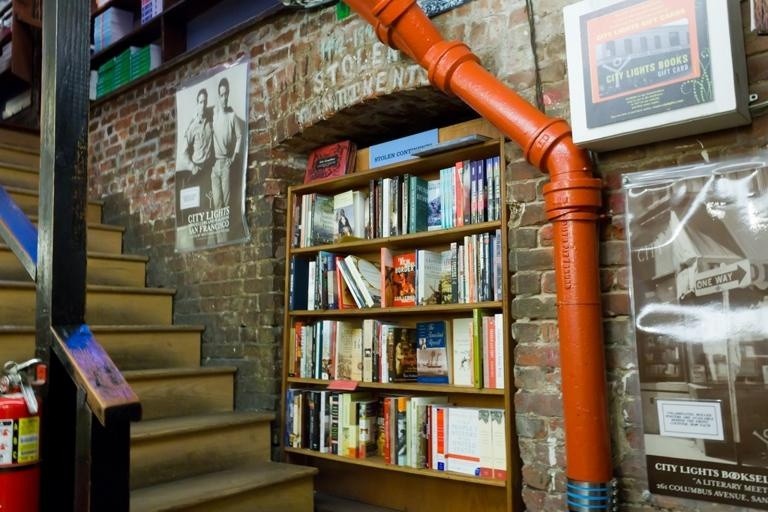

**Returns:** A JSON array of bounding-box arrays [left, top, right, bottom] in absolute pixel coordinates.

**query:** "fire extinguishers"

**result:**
[[0, 357, 46, 512]]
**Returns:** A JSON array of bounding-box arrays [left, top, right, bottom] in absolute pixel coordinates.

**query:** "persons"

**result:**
[[182, 78, 242, 245], [182, 88, 234, 248]]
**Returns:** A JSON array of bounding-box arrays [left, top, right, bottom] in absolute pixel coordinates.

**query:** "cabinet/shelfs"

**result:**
[[0, 0, 41, 128], [88, 1, 290, 110], [282, 141, 518, 512]]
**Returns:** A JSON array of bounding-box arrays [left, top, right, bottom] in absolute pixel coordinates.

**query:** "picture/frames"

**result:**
[[560, 0, 754, 156]]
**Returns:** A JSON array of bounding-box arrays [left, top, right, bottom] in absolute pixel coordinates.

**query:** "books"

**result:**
[[295, 157, 501, 247], [288, 230, 504, 389], [89, 0, 164, 99], [409, 133, 493, 157], [302, 140, 357, 185], [287, 389, 509, 482]]
